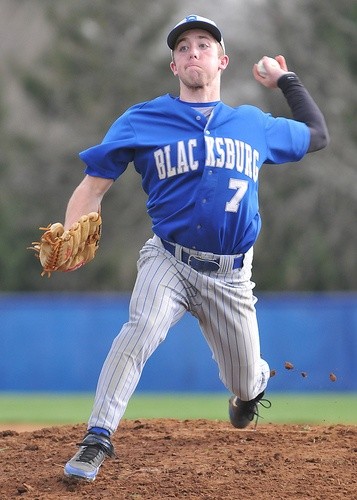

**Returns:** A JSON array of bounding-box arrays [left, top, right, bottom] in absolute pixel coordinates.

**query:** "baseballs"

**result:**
[[258, 55, 280, 78]]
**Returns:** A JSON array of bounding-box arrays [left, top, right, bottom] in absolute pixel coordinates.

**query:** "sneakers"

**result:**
[[229, 385, 271, 428], [64, 427, 119, 482]]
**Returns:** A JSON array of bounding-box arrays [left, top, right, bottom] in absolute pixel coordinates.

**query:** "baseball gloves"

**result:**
[[27, 210, 104, 276]]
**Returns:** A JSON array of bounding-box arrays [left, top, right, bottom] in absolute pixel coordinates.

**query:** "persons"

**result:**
[[39, 14, 330, 490]]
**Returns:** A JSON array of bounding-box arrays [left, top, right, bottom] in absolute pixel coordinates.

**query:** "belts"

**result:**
[[161, 238, 243, 272]]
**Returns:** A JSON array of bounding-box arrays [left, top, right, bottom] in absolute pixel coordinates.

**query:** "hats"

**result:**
[[167, 15, 225, 56]]
